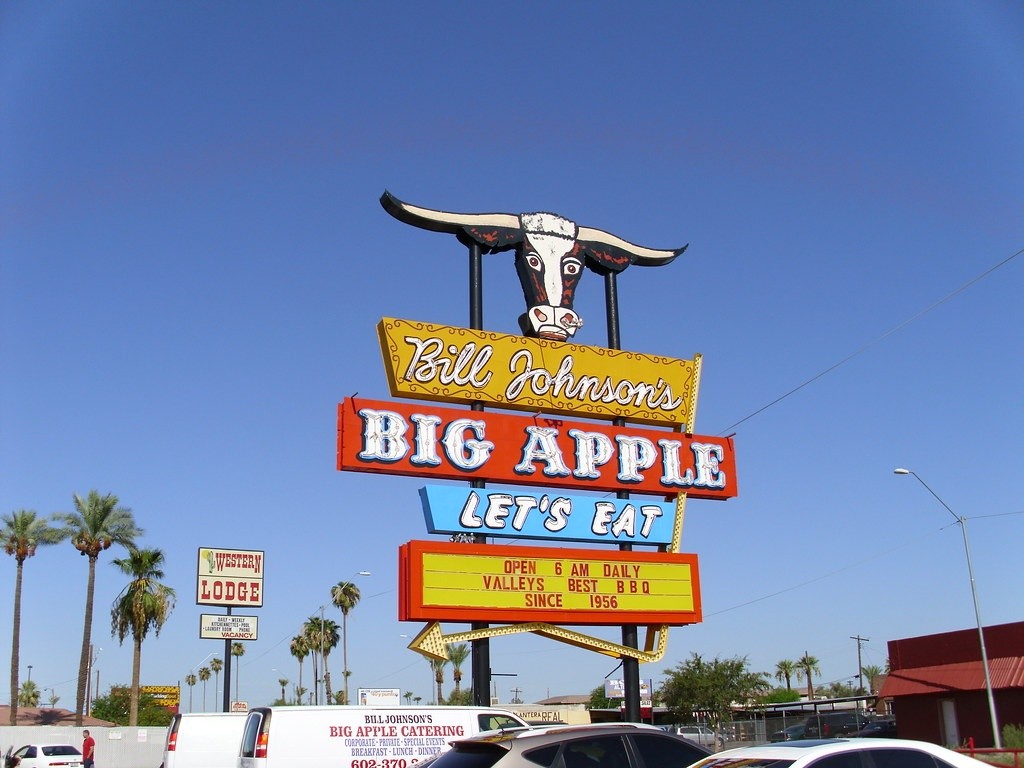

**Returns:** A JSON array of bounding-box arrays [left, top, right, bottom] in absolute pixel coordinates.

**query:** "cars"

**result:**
[[415, 708, 715, 768], [5, 745, 87, 767], [651, 723, 676, 734], [687, 736, 998, 768], [846, 717, 896, 737], [770, 719, 808, 744]]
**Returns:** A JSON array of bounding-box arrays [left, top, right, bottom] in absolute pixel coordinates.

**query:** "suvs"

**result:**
[[803, 712, 873, 740], [673, 724, 726, 747]]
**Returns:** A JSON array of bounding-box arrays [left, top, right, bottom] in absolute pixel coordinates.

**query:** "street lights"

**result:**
[[25, 664, 33, 707], [188, 652, 220, 713], [320, 569, 372, 704], [43, 687, 55, 709], [399, 634, 436, 706], [892, 468, 1001, 750]]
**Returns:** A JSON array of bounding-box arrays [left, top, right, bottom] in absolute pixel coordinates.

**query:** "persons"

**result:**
[[82, 730, 95, 768]]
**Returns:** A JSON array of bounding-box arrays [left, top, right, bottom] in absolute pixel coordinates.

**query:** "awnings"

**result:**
[[878, 620, 1024, 698], [590, 694, 877, 713]]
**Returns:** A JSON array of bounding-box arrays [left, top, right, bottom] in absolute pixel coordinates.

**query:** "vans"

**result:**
[[236, 703, 532, 768], [158, 711, 256, 767]]
